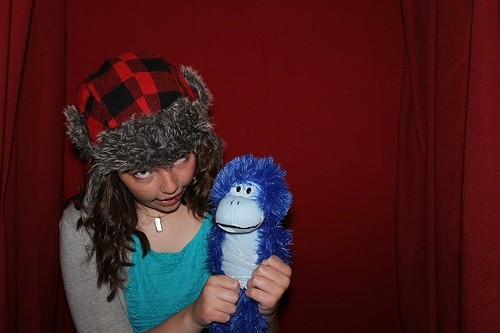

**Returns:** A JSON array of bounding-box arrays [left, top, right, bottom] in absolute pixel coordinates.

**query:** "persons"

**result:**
[[58, 47, 293, 333]]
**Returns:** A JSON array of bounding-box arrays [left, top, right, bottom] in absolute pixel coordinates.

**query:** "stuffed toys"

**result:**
[[201, 152, 297, 333]]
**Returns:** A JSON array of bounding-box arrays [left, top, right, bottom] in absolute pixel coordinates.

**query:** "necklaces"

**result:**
[[132, 204, 174, 234]]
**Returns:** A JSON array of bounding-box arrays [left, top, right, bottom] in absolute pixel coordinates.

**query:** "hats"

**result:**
[[60, 51, 225, 224]]
